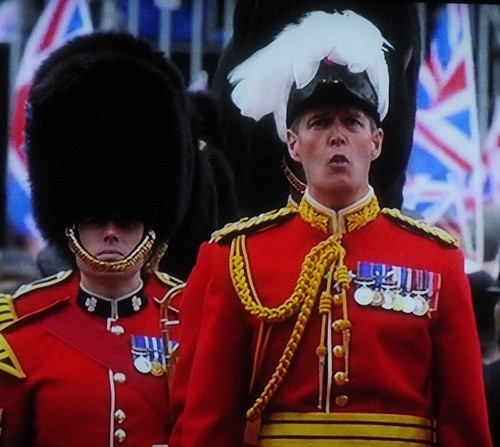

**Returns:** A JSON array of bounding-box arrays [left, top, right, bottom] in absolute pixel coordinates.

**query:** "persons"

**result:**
[[168, 10, 492, 447], [0, 29, 186, 447]]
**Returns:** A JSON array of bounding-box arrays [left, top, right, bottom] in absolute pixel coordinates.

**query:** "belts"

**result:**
[[256, 411, 436, 447]]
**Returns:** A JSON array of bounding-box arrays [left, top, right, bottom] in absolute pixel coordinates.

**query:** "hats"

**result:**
[[285, 67, 378, 124], [22, 26, 195, 271]]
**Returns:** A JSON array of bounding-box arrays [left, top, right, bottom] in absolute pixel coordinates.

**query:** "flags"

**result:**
[[0, 0, 500, 274]]
